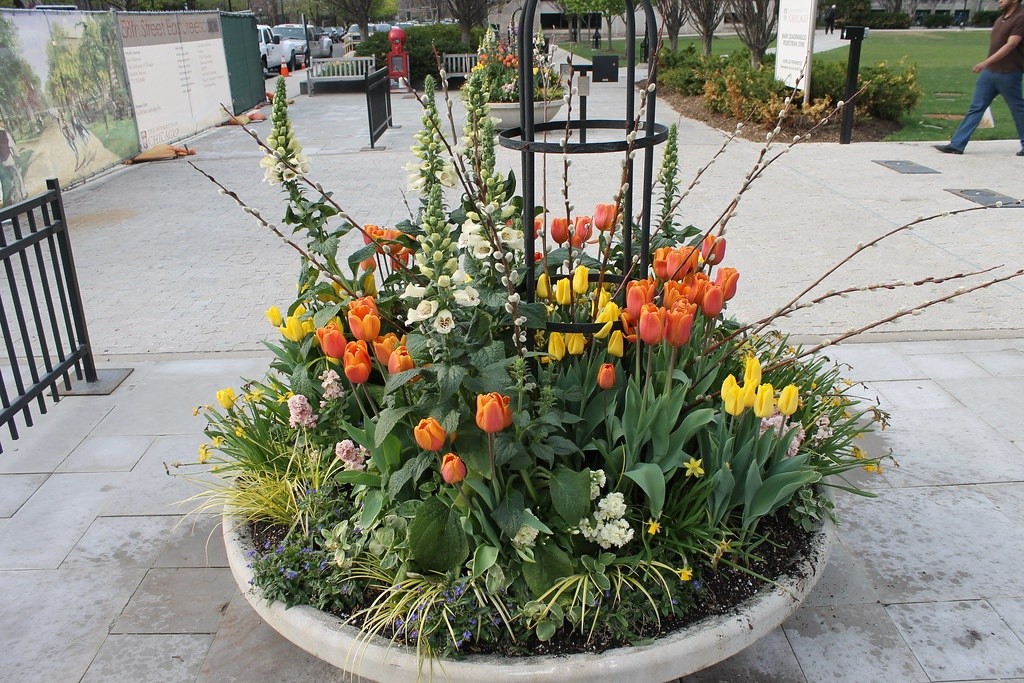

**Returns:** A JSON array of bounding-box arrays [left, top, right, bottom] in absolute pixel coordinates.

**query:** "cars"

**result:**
[[335, 27, 347, 43], [315, 27, 334, 59], [342, 19, 461, 51], [256, 24, 282, 79], [322, 27, 340, 44], [272, 23, 322, 70]]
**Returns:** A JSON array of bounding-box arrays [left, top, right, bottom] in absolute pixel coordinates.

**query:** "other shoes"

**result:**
[[934, 145, 963, 154], [1016, 151, 1024, 156]]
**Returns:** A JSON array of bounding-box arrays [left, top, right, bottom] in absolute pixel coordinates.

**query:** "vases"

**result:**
[[462, 99, 565, 129], [211, 464, 834, 683]]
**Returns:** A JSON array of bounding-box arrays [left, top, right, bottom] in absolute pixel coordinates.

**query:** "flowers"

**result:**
[[167, 51, 1024, 644], [459, 19, 576, 102]]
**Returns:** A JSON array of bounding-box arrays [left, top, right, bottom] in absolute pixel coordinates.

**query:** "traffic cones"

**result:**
[[301, 56, 306, 70], [280, 55, 292, 77]]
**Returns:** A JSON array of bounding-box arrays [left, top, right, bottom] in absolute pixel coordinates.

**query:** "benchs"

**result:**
[[306, 54, 376, 97], [441, 52, 481, 90]]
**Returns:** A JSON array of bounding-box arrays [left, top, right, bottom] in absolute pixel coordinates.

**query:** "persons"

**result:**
[[936, 0, 1024, 156], [824, 4, 836, 35]]
